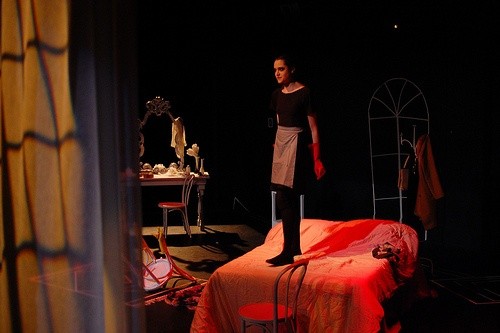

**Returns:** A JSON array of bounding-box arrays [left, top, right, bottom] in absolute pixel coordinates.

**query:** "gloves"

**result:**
[[312, 143, 326, 180]]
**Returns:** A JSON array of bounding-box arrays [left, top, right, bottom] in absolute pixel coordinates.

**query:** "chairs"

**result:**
[[156, 174, 194, 239], [239, 257, 309, 332], [119, 224, 198, 294]]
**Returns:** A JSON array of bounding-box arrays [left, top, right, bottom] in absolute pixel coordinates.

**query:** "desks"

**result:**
[[127, 95, 210, 232]]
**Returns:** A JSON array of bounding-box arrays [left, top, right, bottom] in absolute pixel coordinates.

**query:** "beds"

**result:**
[[189, 217, 420, 332]]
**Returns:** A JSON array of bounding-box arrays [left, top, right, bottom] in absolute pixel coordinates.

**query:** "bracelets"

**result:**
[[313, 156, 321, 163]]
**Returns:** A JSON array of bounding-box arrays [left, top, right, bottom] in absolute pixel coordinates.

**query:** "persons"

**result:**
[[265, 55, 327, 266]]
